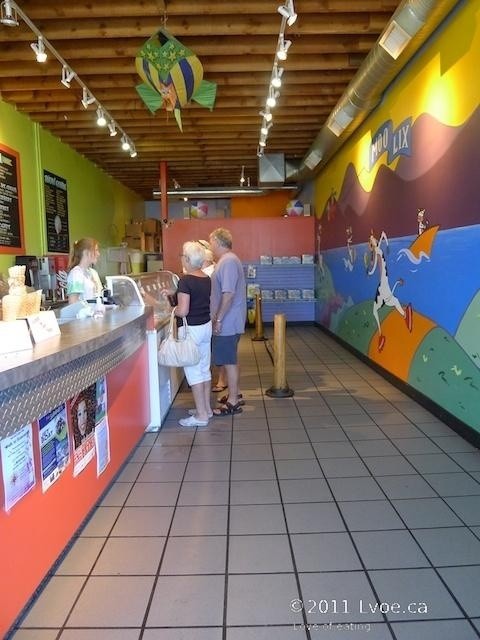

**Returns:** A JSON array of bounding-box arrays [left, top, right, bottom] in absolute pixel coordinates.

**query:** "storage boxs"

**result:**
[[123, 218, 163, 252]]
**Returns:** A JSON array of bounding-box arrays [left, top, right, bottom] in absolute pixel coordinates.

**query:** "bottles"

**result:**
[[94, 296, 104, 317]]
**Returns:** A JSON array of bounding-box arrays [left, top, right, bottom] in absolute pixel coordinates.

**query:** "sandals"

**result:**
[[217, 394, 245, 405], [213, 401, 242, 416]]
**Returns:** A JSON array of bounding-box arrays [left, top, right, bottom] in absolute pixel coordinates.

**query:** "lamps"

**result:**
[[5, 0, 302, 157]]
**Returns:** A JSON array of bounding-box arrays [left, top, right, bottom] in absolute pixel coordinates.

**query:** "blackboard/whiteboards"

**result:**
[[44, 170, 70, 253], [0, 141, 25, 254]]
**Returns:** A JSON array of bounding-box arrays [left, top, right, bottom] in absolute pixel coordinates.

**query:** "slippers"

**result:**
[[211, 385, 227, 392], [188, 409, 213, 419], [178, 415, 209, 427]]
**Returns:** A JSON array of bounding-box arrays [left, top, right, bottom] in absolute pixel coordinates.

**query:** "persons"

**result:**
[[160, 242, 214, 428], [66, 237, 103, 305], [208, 227, 245, 417], [196, 240, 228, 392], [69, 390, 99, 448]]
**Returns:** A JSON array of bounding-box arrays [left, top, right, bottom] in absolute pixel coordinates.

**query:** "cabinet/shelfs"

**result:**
[[245, 264, 321, 302]]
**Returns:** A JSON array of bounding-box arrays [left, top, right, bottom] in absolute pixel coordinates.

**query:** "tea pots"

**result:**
[[56, 288, 67, 302]]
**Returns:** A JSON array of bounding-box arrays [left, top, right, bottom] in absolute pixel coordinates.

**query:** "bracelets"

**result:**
[[216, 319, 222, 323]]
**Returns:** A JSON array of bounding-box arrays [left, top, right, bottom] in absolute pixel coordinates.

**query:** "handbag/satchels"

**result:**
[[158, 335, 200, 367]]
